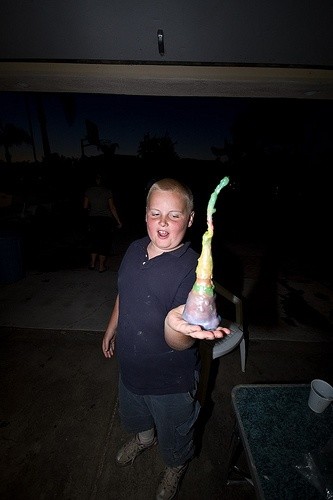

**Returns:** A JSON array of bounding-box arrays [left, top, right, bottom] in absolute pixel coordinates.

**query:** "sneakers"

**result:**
[[113, 433, 157, 468], [156, 462, 187, 500]]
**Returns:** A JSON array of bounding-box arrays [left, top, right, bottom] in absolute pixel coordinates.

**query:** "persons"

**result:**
[[102, 179, 230, 500], [83, 174, 122, 273]]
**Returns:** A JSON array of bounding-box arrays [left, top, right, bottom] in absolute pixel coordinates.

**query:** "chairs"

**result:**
[[194, 280, 246, 408]]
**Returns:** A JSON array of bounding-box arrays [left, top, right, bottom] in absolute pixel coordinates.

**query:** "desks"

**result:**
[[224, 384, 333, 500]]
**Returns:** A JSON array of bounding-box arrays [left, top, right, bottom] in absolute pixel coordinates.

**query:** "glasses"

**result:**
[[147, 208, 191, 222]]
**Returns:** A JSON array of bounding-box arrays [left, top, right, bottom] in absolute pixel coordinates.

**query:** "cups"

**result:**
[[307, 378, 333, 415]]
